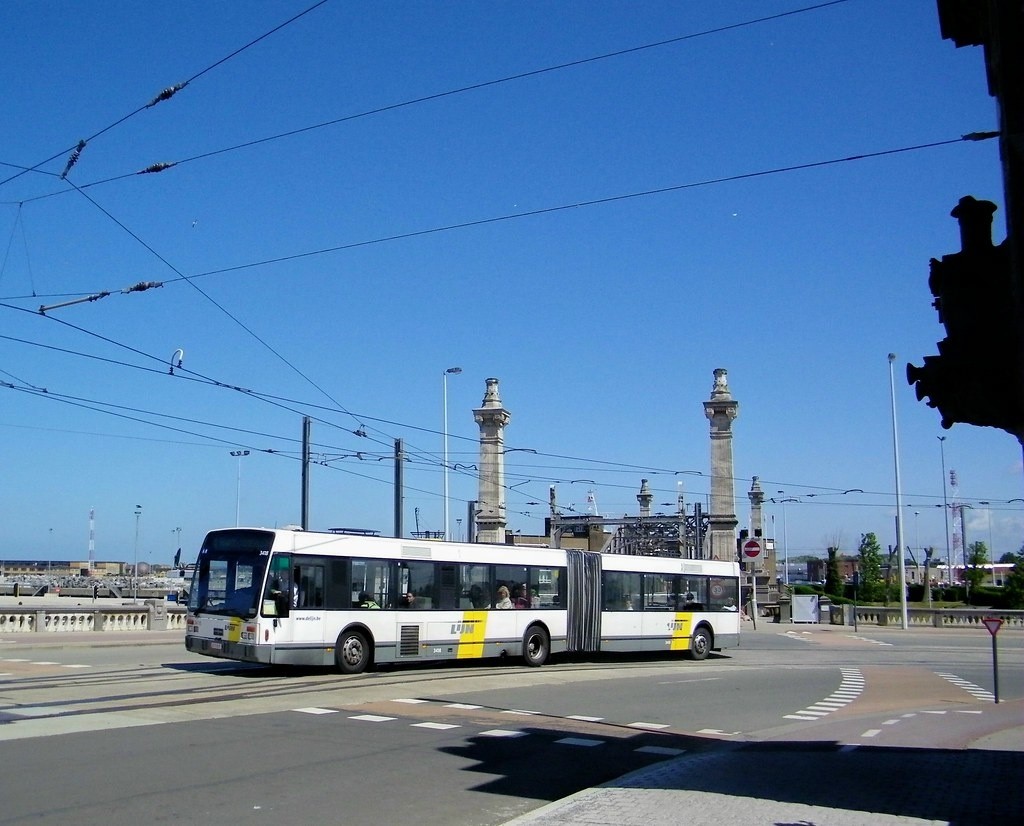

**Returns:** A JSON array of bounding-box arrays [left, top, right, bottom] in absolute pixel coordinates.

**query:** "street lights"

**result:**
[[936, 436, 952, 587], [133, 505, 143, 605], [980, 501, 995, 587], [888, 352, 909, 629], [915, 511, 920, 585], [229, 451, 252, 527], [778, 490, 788, 585], [443, 368, 462, 542]]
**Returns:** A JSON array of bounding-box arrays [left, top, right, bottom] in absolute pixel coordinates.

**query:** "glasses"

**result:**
[[407, 596, 413, 598]]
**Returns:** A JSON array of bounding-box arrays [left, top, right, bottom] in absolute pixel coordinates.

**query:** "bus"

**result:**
[[173, 526, 741, 675]]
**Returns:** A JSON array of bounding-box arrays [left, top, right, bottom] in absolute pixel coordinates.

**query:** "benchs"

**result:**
[[414, 597, 432, 608]]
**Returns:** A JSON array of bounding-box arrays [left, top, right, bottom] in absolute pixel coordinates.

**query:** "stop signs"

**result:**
[[744, 541, 761, 558]]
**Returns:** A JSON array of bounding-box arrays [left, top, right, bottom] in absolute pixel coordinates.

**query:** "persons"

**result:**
[[722, 597, 737, 611], [358, 591, 380, 609], [683, 593, 697, 610], [624, 600, 633, 610], [271, 568, 298, 607], [406, 591, 420, 609], [468, 583, 540, 609]]
